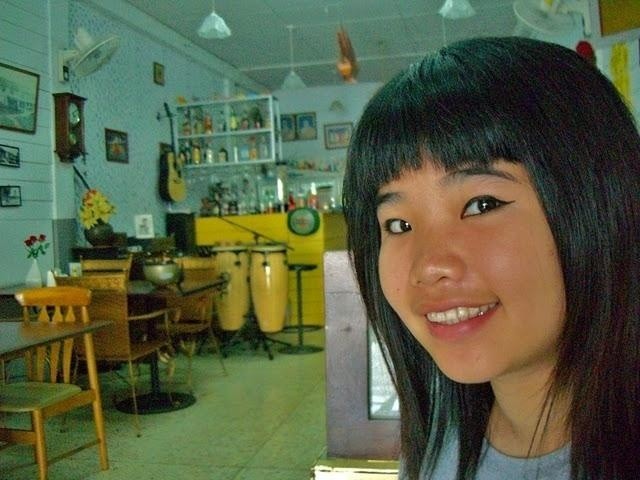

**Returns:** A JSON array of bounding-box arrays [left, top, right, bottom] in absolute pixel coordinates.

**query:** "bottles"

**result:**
[[183, 134, 268, 165], [45, 268, 58, 288], [179, 103, 272, 137], [213, 179, 318, 215]]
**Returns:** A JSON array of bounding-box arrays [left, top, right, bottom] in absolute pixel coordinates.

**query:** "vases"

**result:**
[[84, 223, 114, 247]]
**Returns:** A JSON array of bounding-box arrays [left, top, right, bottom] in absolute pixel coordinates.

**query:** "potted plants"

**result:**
[[143, 231, 181, 286]]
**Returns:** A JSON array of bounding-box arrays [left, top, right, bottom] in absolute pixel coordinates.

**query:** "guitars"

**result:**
[[161, 102, 185, 202]]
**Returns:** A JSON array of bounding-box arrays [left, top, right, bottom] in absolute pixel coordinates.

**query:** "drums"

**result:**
[[210, 244, 250, 332], [249, 243, 289, 333]]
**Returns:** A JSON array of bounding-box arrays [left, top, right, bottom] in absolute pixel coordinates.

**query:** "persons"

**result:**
[[341, 37, 640, 479]]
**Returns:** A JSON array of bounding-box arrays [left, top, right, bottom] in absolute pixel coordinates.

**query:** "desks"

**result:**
[[3, 278, 227, 414], [2, 318, 117, 361]]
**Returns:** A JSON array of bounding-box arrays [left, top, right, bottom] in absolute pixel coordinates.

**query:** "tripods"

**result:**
[[203, 309, 293, 359]]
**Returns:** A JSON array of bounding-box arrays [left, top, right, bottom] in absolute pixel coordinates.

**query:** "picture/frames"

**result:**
[[0, 144, 21, 170], [1, 184, 22, 209], [103, 126, 130, 166], [323, 121, 357, 149], [153, 59, 166, 86], [273, 113, 296, 142], [296, 110, 318, 143], [134, 214, 153, 239], [0, 62, 41, 136]]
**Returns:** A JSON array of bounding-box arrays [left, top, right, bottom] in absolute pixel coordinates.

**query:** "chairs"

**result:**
[[53, 270, 174, 439], [0, 283, 106, 478], [80, 252, 135, 280], [145, 265, 228, 393]]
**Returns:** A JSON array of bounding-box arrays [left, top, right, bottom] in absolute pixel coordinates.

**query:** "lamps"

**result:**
[[274, 26, 311, 95], [438, 1, 476, 22], [196, 1, 234, 39]]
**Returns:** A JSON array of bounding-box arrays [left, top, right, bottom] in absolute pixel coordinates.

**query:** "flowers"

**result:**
[[23, 234, 50, 260], [74, 187, 117, 227]]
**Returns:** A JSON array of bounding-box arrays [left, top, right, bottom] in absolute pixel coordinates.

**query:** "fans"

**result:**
[[56, 22, 125, 88], [512, 0, 596, 41]]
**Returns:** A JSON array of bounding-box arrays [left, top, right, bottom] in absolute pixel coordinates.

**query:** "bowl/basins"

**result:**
[[141, 263, 181, 289]]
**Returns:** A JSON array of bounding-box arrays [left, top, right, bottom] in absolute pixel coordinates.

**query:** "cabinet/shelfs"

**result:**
[[176, 93, 284, 173]]
